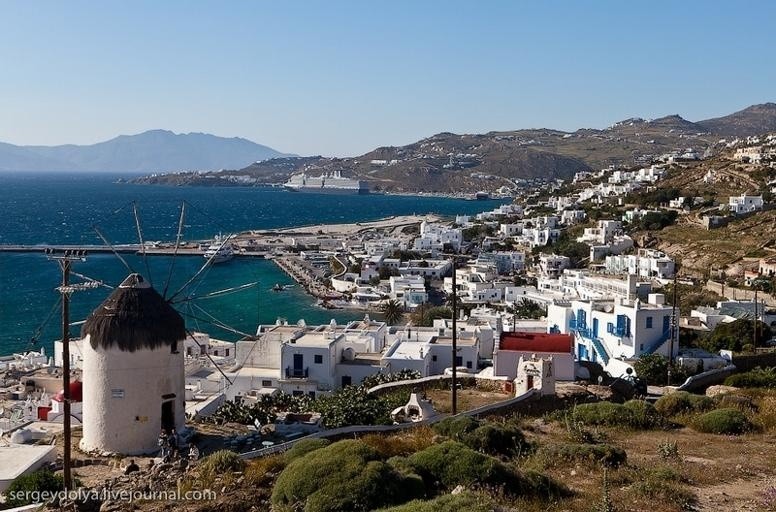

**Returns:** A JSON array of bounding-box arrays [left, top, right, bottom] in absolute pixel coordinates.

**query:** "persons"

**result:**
[[124, 429, 200, 476]]
